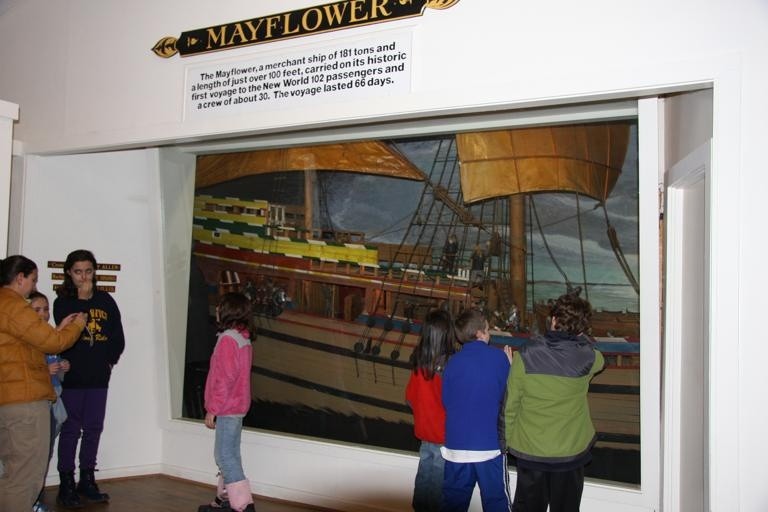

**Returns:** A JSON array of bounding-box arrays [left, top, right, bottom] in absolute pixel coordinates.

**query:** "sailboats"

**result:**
[[190, 120, 639, 455]]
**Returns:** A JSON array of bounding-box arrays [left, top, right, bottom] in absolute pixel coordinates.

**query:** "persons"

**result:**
[[442, 234, 458, 273], [504, 293, 607, 511], [432, 301, 515, 510], [52, 248, 125, 509], [476, 299, 522, 333], [191, 291, 259, 512], [466, 244, 485, 294], [0, 253, 90, 512], [404, 307, 454, 511], [24, 290, 72, 511]]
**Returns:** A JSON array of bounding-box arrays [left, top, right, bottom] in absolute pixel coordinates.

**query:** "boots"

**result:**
[[56, 473, 80, 504], [198, 472, 255, 512], [77, 471, 110, 502]]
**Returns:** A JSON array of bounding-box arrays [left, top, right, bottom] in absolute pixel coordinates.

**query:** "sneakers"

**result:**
[[33, 501, 49, 512]]
